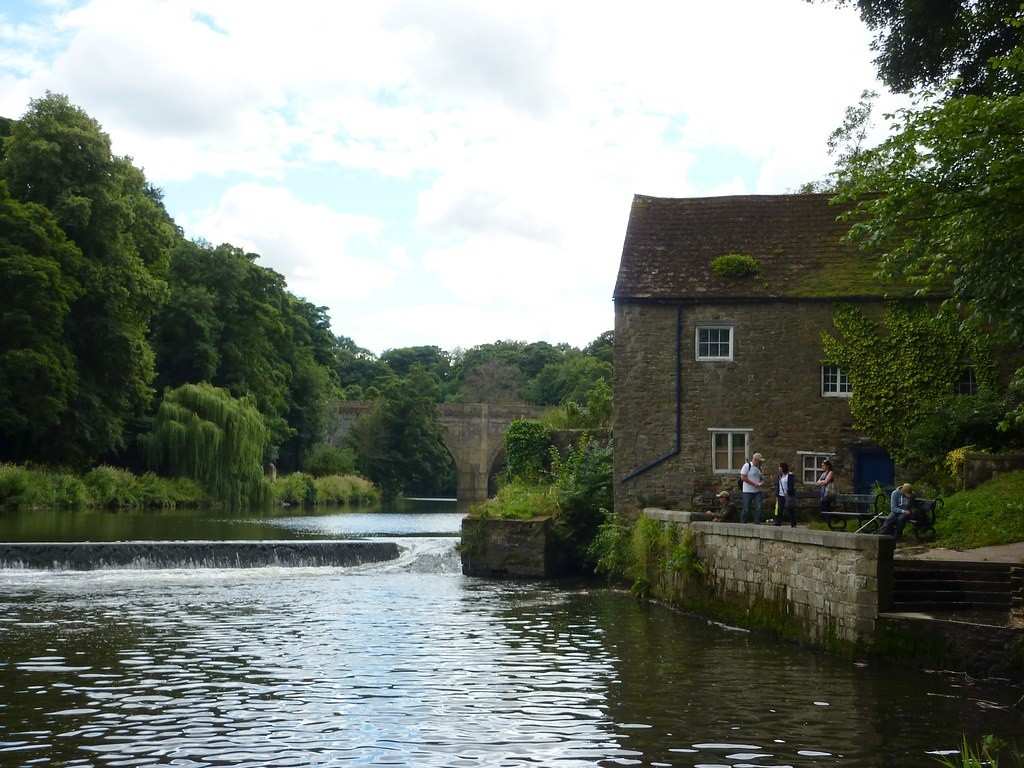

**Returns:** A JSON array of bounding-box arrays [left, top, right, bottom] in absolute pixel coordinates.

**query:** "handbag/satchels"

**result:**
[[824, 481, 838, 499], [785, 496, 797, 508], [774, 497, 778, 516]]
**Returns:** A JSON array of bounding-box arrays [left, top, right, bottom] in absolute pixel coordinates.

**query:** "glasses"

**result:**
[[823, 459, 828, 462]]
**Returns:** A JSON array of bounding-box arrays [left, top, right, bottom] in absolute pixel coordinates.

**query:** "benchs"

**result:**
[[703, 490, 834, 515], [878, 498, 944, 540], [821, 492, 886, 534]]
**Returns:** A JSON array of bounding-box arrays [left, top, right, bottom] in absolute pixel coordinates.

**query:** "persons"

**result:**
[[740, 452, 765, 524], [818, 460, 835, 523], [774, 463, 797, 528], [880, 483, 916, 540], [706, 491, 739, 523]]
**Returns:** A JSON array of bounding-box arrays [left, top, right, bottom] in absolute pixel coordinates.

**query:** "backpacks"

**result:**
[[738, 463, 762, 490]]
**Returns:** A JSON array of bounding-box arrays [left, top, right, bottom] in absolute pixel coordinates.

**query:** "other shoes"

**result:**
[[896, 532, 907, 540]]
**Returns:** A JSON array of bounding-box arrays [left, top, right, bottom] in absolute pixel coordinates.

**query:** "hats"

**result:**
[[903, 483, 912, 498], [753, 453, 765, 462], [716, 491, 729, 497]]
